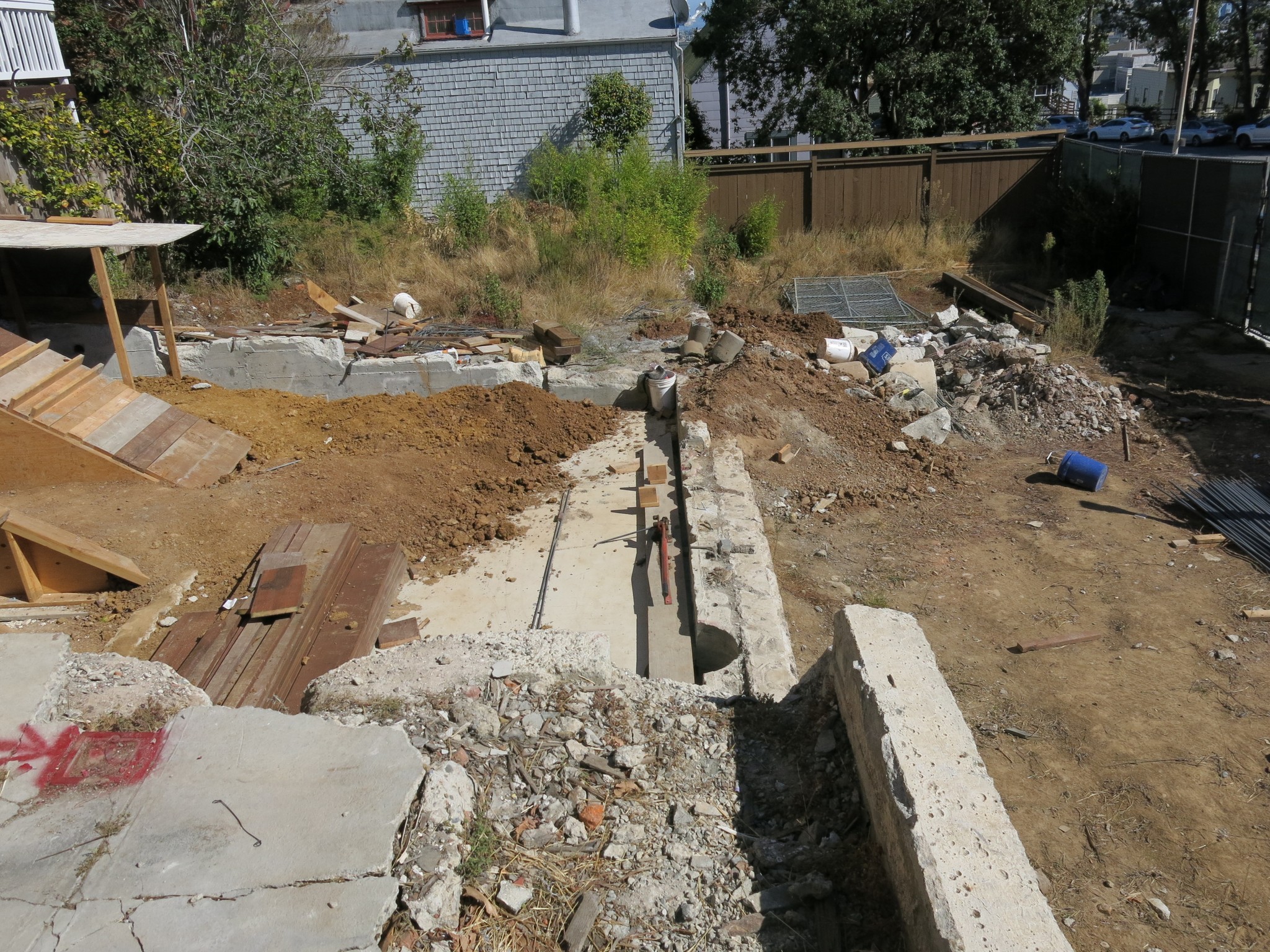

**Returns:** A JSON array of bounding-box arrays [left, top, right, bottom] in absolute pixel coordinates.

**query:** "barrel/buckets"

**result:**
[[392, 292, 422, 319], [648, 370, 676, 412], [816, 337, 855, 363], [1046, 448, 1109, 492], [856, 336, 898, 376]]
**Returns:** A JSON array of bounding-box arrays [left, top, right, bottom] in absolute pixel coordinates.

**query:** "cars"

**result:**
[[1031, 113, 1089, 138], [1160, 119, 1232, 148], [1089, 117, 1156, 142], [1234, 115, 1270, 150]]
